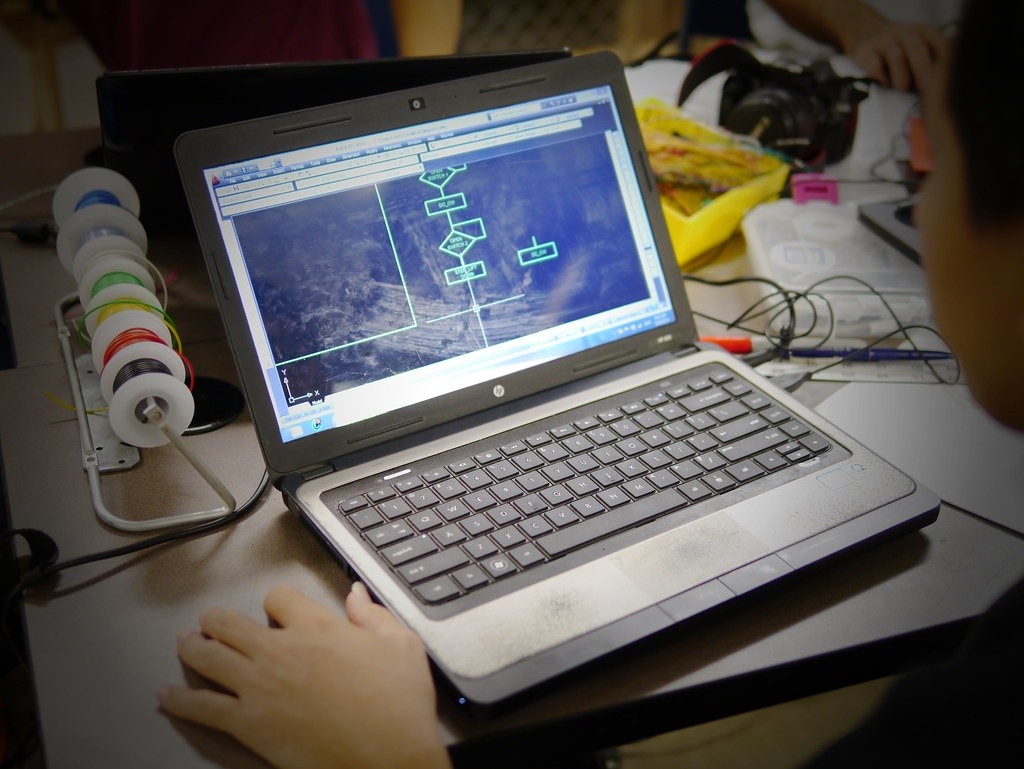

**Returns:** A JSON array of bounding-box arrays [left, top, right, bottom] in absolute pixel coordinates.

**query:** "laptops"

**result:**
[[98, 49, 942, 714]]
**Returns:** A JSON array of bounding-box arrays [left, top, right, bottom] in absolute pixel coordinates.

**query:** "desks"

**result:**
[[1, 54, 1022, 769]]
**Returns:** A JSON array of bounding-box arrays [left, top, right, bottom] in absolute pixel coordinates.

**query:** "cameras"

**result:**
[[720, 52, 866, 162]]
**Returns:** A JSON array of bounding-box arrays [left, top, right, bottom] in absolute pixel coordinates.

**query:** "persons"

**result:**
[[40, 2, 463, 80], [151, 0, 1024, 769], [743, 0, 955, 89]]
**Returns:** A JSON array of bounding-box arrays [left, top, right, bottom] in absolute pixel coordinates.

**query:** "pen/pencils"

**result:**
[[789, 343, 955, 361]]
